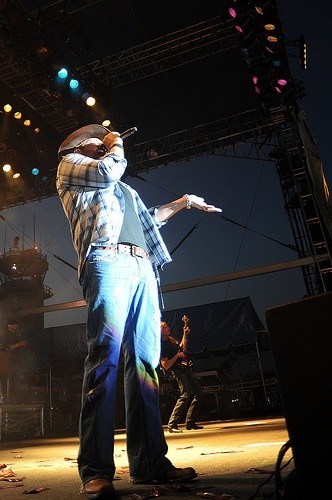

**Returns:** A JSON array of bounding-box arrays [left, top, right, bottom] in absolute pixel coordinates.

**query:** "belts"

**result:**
[[94, 245, 149, 259]]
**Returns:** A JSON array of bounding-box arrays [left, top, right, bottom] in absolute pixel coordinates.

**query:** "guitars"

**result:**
[[171, 315, 193, 370]]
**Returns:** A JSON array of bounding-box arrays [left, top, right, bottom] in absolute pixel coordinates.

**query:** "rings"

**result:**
[[205, 208, 208, 212]]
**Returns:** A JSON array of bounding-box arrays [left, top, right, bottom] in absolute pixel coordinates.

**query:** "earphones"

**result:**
[[8, 325, 10, 326]]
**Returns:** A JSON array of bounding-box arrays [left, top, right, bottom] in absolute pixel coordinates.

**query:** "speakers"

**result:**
[[262, 291, 332, 500]]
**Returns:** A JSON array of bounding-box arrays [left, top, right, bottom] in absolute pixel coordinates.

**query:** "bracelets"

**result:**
[[184, 193, 191, 210], [109, 144, 124, 154]]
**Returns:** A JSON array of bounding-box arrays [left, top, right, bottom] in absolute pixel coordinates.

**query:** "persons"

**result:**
[[57, 125, 222, 500], [2, 319, 27, 368], [159, 315, 204, 433]]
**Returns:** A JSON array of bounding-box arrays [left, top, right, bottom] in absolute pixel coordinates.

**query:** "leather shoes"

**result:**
[[143, 465, 198, 483], [84, 477, 115, 500], [185, 423, 203, 430], [168, 428, 182, 433]]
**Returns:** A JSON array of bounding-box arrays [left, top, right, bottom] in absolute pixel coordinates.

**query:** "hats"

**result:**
[[7, 319, 19, 326], [58, 124, 111, 163]]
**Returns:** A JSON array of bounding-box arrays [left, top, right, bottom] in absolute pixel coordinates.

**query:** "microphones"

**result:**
[[100, 127, 137, 152]]
